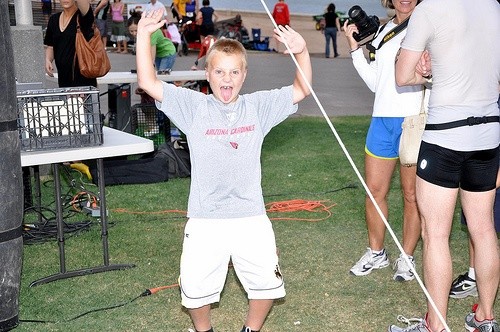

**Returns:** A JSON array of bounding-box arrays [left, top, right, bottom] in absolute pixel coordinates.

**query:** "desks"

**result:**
[[19, 124, 157, 287], [46, 71, 210, 135]]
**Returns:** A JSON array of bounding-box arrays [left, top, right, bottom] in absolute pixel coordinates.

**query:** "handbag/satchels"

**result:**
[[398, 111, 430, 167], [91, 153, 169, 185], [72, 14, 111, 81]]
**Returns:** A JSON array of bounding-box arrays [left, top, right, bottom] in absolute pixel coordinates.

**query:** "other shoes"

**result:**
[[144, 125, 160, 137]]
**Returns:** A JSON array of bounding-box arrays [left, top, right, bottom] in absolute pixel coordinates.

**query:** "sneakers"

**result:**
[[448, 272, 479, 298], [464, 312, 498, 332], [350, 247, 390, 276], [388, 314, 448, 332], [391, 252, 417, 281]]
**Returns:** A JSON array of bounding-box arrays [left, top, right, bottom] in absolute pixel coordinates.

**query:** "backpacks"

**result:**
[[152, 141, 191, 177]]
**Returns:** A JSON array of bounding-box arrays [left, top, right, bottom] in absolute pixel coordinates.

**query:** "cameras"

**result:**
[[347, 5, 380, 42]]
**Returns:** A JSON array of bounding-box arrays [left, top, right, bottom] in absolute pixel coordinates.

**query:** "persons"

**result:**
[[137, 6, 311, 332], [43, 0, 97, 87], [342, 0, 500, 300], [92, 0, 218, 57], [389, 0, 500, 332], [272, 0, 290, 53], [41, 0, 52, 31], [322, 4, 341, 58], [127, 9, 176, 72]]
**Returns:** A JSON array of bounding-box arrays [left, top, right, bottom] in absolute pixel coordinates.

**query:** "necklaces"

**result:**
[[62, 8, 75, 30]]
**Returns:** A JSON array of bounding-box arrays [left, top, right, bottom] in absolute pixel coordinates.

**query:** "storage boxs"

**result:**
[[16, 85, 103, 152], [129, 103, 172, 153]]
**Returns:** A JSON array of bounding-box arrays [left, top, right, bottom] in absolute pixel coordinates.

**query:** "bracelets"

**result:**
[[348, 44, 361, 54], [422, 74, 432, 79]]
